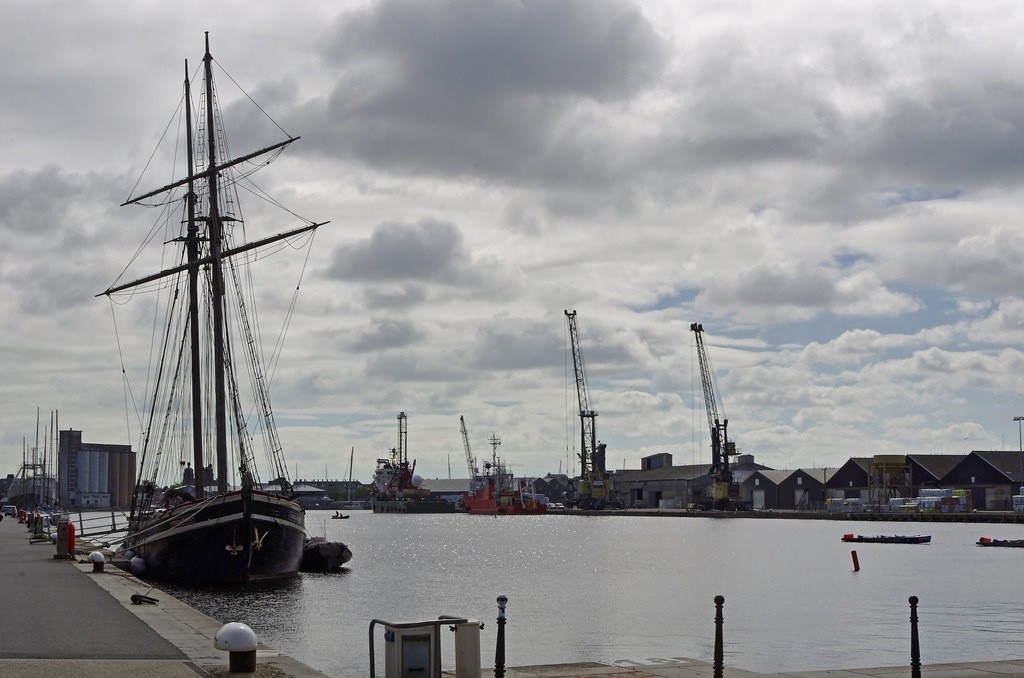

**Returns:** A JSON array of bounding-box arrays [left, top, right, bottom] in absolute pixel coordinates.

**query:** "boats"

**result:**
[[975, 537, 1024, 548], [369, 410, 456, 515], [462, 430, 549, 515], [300, 532, 356, 575], [841, 532, 932, 545]]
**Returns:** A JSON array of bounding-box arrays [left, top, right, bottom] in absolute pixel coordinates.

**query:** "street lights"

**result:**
[[1012, 415, 1024, 487]]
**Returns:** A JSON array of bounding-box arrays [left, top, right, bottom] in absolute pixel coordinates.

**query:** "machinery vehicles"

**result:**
[[560, 308, 616, 510], [686, 322, 755, 512], [459, 413, 496, 497]]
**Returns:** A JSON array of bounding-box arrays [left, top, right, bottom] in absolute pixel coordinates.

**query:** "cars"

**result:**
[[2, 504, 17, 516]]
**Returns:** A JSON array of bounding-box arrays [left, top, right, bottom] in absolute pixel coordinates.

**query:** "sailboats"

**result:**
[[21, 404, 73, 526], [93, 26, 338, 595]]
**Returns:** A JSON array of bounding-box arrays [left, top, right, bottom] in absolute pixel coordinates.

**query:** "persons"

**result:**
[[183, 462, 213, 482], [336, 511, 343, 518]]
